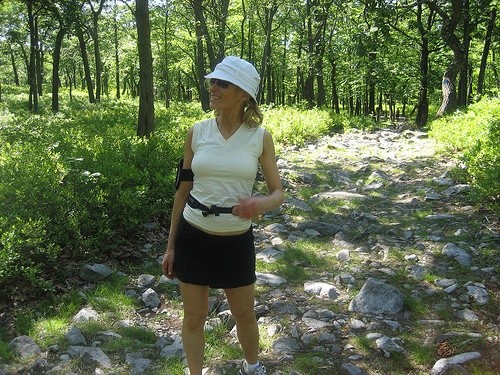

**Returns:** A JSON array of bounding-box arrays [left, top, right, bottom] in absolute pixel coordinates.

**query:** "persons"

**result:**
[[162, 56, 285, 375]]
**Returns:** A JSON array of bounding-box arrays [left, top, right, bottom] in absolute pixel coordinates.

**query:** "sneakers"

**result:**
[[239, 360, 267, 375]]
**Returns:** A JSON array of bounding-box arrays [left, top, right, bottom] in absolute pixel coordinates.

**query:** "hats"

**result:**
[[204, 56, 261, 104]]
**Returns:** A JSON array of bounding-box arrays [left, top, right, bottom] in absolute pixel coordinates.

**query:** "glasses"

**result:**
[[210, 78, 234, 89]]
